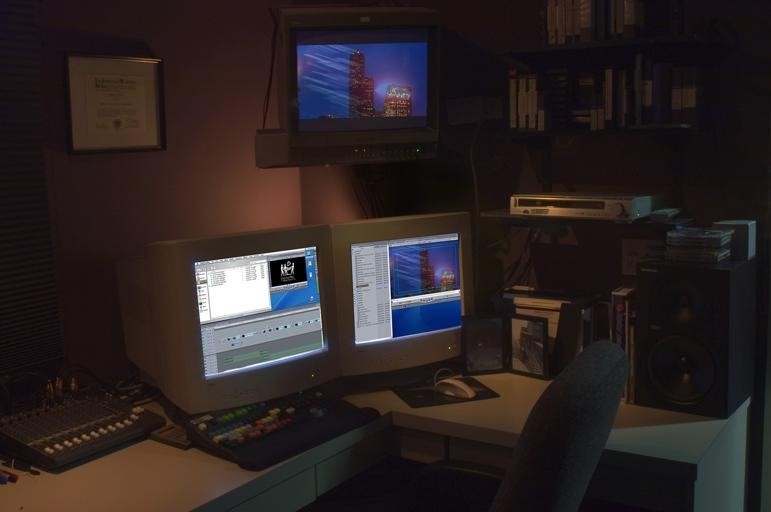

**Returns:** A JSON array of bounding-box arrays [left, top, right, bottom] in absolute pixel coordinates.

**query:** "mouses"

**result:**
[[433, 377, 477, 399]]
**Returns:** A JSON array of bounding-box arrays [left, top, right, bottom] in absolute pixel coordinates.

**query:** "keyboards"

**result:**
[[186, 387, 380, 473]]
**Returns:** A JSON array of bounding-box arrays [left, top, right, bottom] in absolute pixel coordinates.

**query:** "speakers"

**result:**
[[635, 252, 757, 419]]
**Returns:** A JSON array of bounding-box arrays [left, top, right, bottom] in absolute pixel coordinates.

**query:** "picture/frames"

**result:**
[[63, 51, 166, 154]]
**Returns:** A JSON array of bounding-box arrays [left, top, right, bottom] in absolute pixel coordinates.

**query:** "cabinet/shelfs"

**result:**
[[474, 40, 713, 244]]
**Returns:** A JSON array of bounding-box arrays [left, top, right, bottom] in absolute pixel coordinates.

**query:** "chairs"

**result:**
[[488, 341, 630, 511]]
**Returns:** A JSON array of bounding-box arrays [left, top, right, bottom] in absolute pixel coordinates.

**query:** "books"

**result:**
[[508, 0, 703, 133], [609, 284, 636, 403]]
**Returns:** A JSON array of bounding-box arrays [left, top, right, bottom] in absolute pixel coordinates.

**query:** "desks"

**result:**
[[1, 369, 755, 511]]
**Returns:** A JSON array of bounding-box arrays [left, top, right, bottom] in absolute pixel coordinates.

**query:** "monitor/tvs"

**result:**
[[117, 222, 339, 414], [331, 208, 476, 376], [254, 11, 442, 170]]
